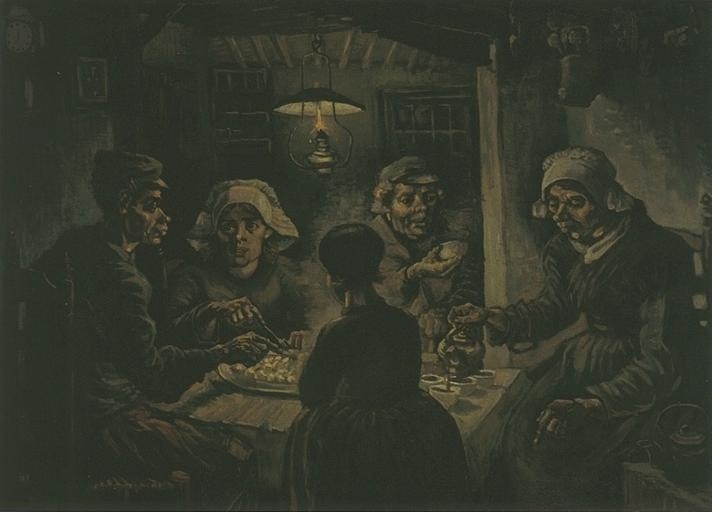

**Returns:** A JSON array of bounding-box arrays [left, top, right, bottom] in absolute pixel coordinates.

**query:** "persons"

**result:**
[[68, 144, 697, 510]]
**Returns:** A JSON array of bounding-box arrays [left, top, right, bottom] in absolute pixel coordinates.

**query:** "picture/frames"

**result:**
[[72, 54, 110, 101]]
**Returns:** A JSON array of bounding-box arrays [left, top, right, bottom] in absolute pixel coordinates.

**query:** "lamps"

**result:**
[[271, 10, 370, 179]]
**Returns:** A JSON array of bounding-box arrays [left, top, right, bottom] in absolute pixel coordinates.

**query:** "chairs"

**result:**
[[641, 190, 712, 383], [0, 229, 194, 512]]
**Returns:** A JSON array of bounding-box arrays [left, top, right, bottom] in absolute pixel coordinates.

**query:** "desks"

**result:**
[[621, 460, 712, 512]]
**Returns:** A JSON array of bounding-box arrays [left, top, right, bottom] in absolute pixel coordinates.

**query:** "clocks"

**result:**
[[0, 16, 42, 58]]
